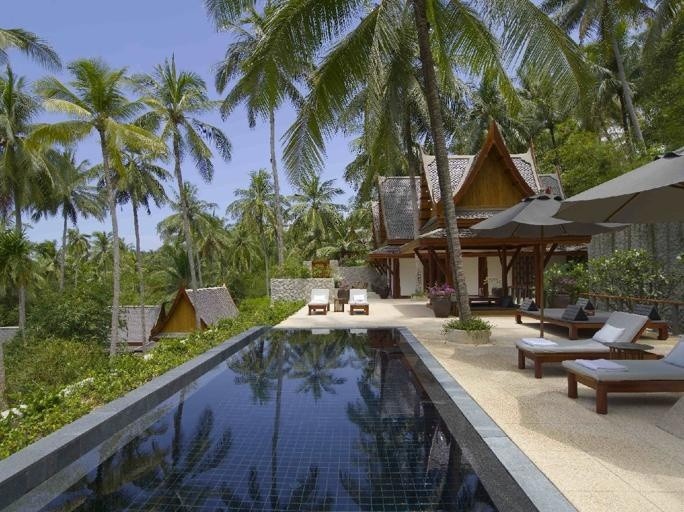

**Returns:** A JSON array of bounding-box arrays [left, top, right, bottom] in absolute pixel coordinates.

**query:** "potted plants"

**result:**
[[543, 260, 592, 308]]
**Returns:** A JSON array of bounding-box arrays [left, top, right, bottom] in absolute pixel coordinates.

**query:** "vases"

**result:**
[[431, 296, 452, 317]]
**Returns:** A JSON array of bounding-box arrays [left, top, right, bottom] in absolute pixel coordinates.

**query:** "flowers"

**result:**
[[425, 282, 456, 296]]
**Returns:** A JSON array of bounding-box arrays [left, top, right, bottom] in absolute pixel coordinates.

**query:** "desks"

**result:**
[[515, 308, 668, 340]]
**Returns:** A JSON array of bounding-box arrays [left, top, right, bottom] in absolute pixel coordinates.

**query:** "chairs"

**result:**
[[308, 288, 369, 315], [515, 308, 683, 415]]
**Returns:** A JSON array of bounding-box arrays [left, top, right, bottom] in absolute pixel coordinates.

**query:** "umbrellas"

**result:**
[[471, 191, 634, 337], [548, 149, 684, 236]]
